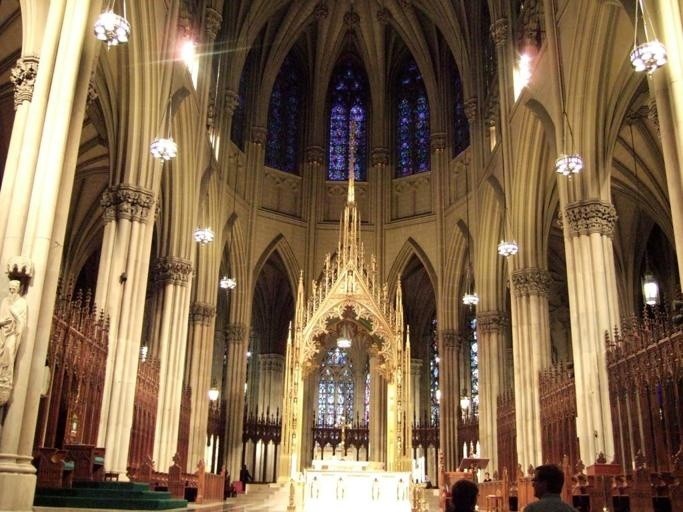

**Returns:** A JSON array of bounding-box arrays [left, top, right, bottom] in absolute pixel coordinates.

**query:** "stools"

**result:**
[[104, 473, 119, 481]]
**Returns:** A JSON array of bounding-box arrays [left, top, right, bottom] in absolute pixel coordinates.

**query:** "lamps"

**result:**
[[629, 124, 660, 308], [461, 388, 470, 410], [629, 0, 668, 79], [220, 154, 237, 291], [151, 0, 178, 166], [552, 0, 582, 180], [462, 168, 480, 311], [193, 128, 214, 247], [93, 0, 131, 51], [498, 110, 518, 258]]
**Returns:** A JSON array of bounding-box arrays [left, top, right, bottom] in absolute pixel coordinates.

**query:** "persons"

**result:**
[[484, 472, 491, 482], [522, 464, 579, 511], [0, 279, 29, 408], [239, 464, 252, 482], [425, 474, 432, 488], [447, 479, 479, 511]]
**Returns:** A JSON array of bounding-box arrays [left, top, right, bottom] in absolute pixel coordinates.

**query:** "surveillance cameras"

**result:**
[[121, 272, 127, 282]]
